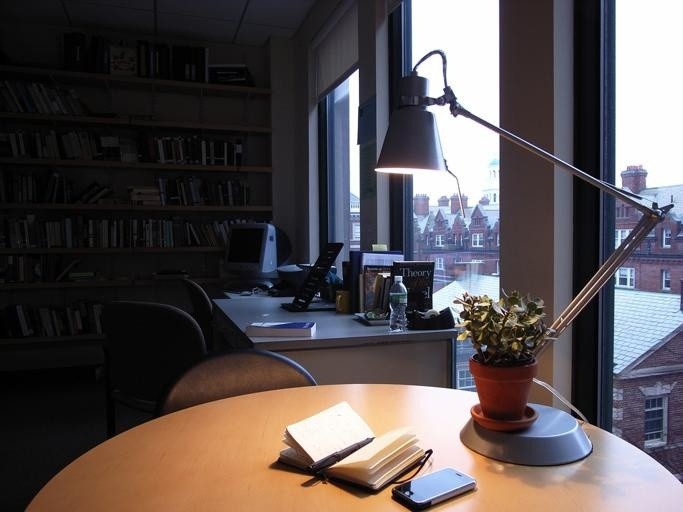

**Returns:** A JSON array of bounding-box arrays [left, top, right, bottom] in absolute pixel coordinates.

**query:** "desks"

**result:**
[[214, 288, 456, 390], [19, 379, 682, 512]]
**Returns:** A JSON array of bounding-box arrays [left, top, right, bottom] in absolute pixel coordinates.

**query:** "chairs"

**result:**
[[159, 347, 316, 413], [181, 278, 215, 331], [92, 298, 204, 439]]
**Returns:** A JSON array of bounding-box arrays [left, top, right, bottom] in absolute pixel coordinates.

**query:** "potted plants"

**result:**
[[446, 284, 564, 433]]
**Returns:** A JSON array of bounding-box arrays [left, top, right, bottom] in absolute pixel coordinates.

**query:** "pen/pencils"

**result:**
[[305, 435, 376, 477]]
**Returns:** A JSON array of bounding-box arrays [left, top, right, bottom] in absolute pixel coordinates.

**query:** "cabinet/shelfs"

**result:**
[[0, 33, 273, 344]]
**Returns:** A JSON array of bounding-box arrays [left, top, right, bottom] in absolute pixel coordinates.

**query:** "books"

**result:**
[[243, 319, 317, 339], [2, 2, 277, 345], [340, 249, 435, 315], [275, 399, 429, 495]]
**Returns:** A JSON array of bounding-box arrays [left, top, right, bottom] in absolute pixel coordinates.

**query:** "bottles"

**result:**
[[388, 275, 408, 331]]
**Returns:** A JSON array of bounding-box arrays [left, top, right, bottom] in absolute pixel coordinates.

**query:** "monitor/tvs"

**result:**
[[223, 222, 294, 291]]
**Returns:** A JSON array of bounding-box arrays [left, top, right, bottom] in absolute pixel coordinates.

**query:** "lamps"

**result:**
[[373, 49, 677, 469]]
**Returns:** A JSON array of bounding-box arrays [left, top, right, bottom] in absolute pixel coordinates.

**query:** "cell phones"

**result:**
[[390, 467, 477, 512]]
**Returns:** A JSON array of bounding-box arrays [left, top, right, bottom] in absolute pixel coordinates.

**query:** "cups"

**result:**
[[335, 290, 350, 312]]
